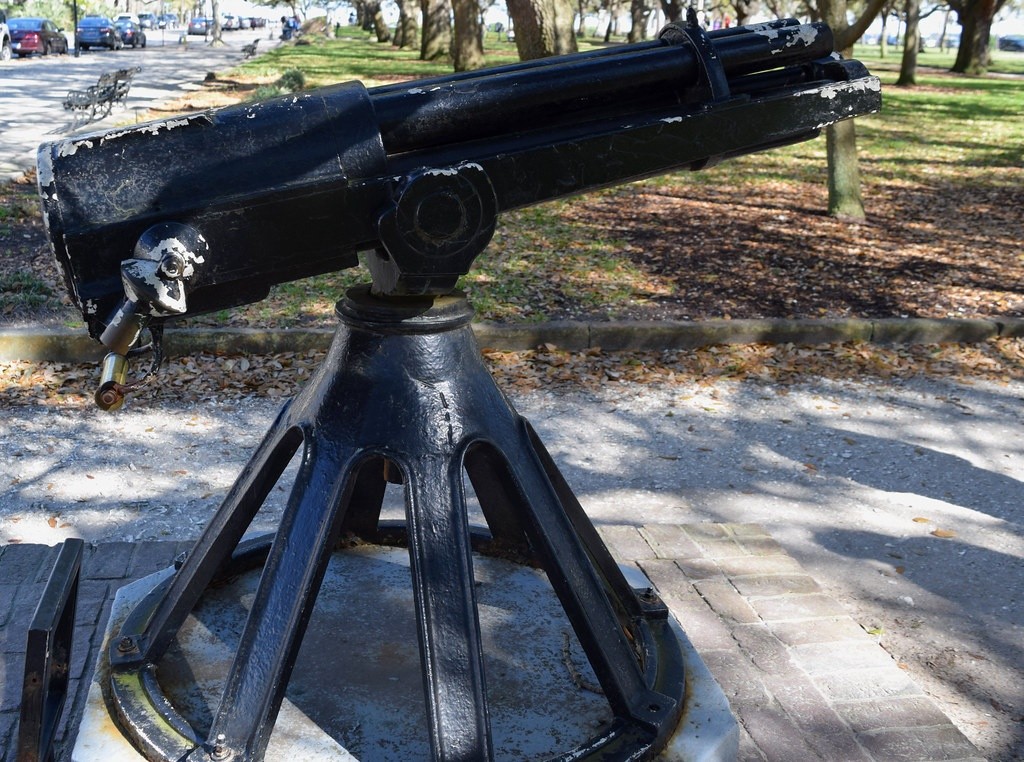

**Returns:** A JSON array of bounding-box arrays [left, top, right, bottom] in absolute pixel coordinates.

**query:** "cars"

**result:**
[[76, 16, 124, 51], [187, 16, 214, 36], [112, 11, 180, 49], [219, 12, 269, 31], [6, 17, 68, 57], [858, 32, 962, 50], [999, 34, 1024, 52], [0, 9, 12, 64]]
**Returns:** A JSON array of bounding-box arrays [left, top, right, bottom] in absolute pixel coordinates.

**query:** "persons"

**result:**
[[251, 12, 300, 41], [322, 11, 356, 37], [696, 9, 731, 32]]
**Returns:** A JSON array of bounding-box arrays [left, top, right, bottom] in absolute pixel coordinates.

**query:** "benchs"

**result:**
[[92, 67, 143, 116], [61, 72, 119, 131], [240, 39, 261, 59]]
